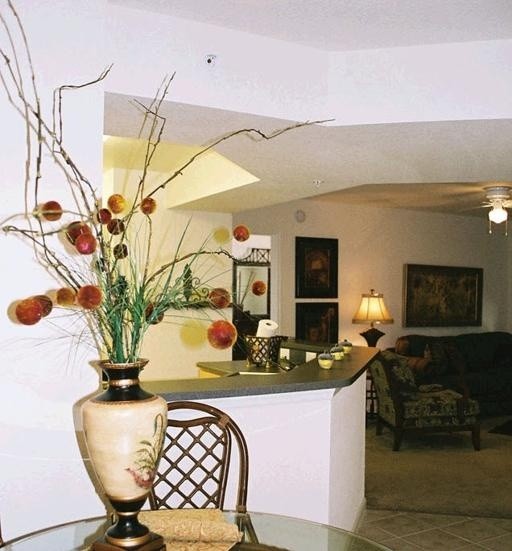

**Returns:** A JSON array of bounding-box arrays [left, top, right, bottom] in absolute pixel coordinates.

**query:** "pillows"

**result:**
[[380, 350, 418, 392]]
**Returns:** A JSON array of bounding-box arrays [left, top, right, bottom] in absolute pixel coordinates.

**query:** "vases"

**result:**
[[82, 360, 167, 548]]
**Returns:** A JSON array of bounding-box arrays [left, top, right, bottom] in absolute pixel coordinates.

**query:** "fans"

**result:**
[[458, 185, 512, 213]]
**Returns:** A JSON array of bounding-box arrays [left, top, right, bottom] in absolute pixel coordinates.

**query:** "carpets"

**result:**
[[365, 414, 512, 519], [488, 420, 512, 435]]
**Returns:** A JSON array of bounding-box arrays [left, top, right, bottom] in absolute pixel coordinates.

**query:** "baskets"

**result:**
[[244, 335, 287, 369]]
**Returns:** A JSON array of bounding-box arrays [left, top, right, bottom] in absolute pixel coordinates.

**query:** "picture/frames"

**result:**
[[295, 236, 338, 298], [295, 302, 338, 344], [402, 264, 484, 328]]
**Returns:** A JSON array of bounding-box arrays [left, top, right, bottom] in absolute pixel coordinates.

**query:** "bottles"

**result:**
[[318, 349, 334, 371], [339, 339, 352, 354], [330, 344, 344, 361]]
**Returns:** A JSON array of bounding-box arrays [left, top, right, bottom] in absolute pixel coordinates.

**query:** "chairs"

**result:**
[[369, 355, 481, 452], [149, 401, 248, 512]]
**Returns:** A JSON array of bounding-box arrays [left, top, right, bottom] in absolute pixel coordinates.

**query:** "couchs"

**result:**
[[394, 331, 512, 416]]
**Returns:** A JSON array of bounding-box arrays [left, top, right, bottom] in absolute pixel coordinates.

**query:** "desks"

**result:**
[[0, 508, 395, 550]]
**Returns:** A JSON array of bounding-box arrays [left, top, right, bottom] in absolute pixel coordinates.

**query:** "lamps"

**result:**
[[352, 288, 394, 347], [488, 205, 508, 236]]
